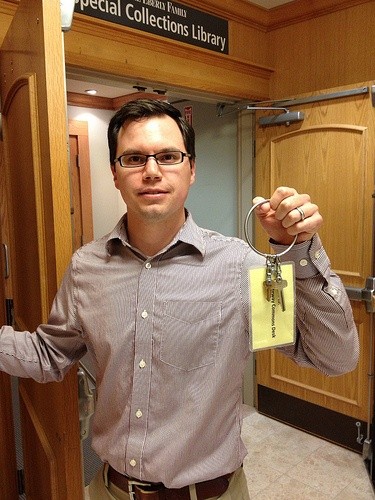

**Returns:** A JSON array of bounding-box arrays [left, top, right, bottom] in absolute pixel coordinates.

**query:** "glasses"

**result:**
[[114, 150, 191, 168]]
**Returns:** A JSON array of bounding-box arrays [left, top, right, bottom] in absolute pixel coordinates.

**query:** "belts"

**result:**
[[103, 461, 234, 500]]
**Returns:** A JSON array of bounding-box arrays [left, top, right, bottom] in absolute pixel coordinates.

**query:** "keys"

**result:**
[[264, 276, 287, 311]]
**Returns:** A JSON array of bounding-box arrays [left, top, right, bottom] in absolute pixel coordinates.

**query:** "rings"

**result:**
[[297, 207, 305, 220]]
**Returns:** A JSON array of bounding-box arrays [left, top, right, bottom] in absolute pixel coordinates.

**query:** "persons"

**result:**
[[0, 98, 360, 500]]
